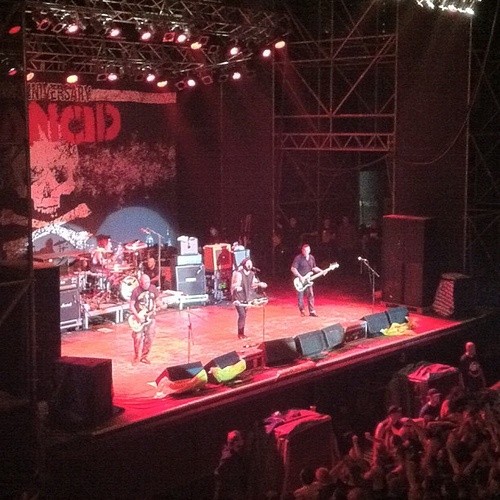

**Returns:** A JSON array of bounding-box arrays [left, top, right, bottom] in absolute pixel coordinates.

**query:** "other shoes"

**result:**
[[309, 312, 318, 317]]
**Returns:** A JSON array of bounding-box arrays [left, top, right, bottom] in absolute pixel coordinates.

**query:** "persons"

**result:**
[[209, 217, 382, 282], [144, 258, 166, 289], [290, 244, 327, 318], [90, 236, 116, 274], [231, 258, 267, 341], [213, 341, 500, 500], [130, 274, 168, 364]]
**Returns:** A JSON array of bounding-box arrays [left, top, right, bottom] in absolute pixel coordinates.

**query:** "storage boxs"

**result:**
[[232, 248, 251, 267], [204, 243, 232, 270], [60, 289, 80, 322], [171, 264, 206, 296]]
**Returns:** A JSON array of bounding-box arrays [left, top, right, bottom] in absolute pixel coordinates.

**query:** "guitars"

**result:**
[[127, 303, 168, 333], [293, 261, 340, 292], [233, 298, 269, 308]]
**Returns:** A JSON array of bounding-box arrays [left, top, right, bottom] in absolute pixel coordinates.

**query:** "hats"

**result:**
[[387, 405, 402, 413], [427, 387, 441, 396]]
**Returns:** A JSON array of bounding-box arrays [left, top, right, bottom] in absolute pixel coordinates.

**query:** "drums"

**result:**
[[91, 274, 107, 290], [123, 251, 134, 265], [106, 275, 140, 302], [74, 271, 92, 290]]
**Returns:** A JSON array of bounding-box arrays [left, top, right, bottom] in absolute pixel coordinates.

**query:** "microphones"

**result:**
[[253, 267, 260, 272], [358, 257, 367, 261]]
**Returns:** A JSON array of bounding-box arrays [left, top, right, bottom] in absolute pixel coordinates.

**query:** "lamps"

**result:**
[[60, 13, 76, 33], [415, 0, 483, 15], [0, 58, 17, 77], [0, 15, 21, 32], [64, 67, 78, 83], [105, 65, 119, 80], [139, 18, 294, 88], [105, 21, 120, 37], [23, 69, 34, 81]]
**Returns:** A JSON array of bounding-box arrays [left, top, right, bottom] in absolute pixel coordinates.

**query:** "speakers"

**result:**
[[257, 337, 297, 368], [442, 273, 475, 319], [52, 355, 113, 429], [384, 305, 409, 325], [204, 244, 232, 270], [294, 330, 326, 357], [380, 215, 438, 306], [172, 265, 206, 295], [60, 289, 79, 322], [34, 263, 61, 402], [321, 323, 345, 350], [361, 312, 390, 338], [158, 351, 243, 390]]
